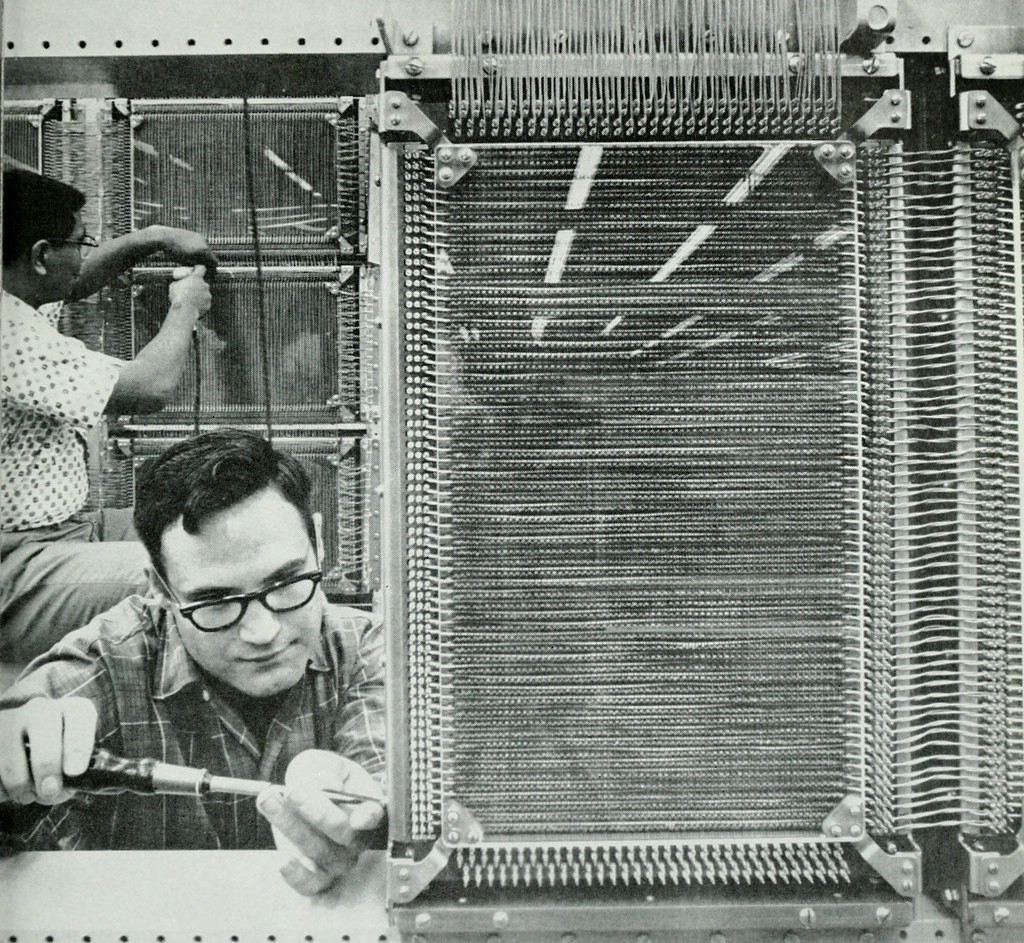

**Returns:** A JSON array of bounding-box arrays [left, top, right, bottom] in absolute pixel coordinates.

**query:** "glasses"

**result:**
[[42, 234, 98, 259], [149, 561, 325, 632]]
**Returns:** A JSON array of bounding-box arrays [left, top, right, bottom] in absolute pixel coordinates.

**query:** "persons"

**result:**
[[0, 169, 217, 661], [0, 429, 389, 896]]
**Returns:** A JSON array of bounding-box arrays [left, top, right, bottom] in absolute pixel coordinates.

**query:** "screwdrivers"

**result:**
[[24, 740, 384, 812]]
